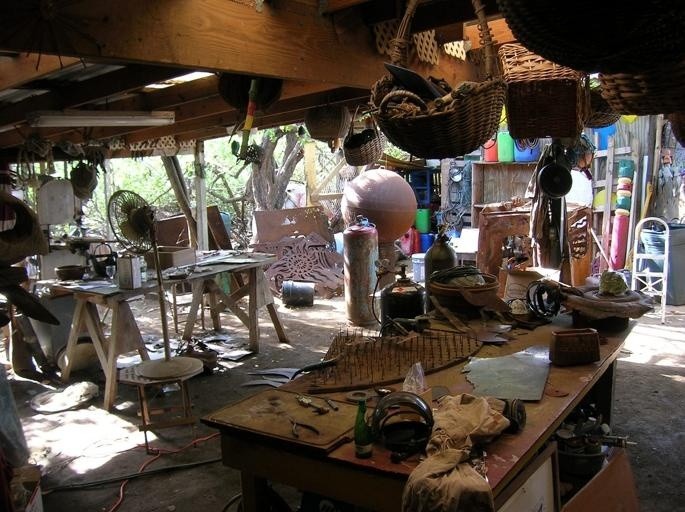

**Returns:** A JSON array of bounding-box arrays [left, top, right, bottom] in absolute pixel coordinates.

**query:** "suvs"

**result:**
[[497, 132, 513, 163], [591, 124, 617, 151], [483, 138, 497, 162], [411, 253, 425, 283], [282, 280, 317, 306], [414, 208, 432, 234], [420, 234, 433, 253], [513, 139, 540, 162]]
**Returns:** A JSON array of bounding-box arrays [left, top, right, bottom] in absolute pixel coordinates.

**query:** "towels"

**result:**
[[640, 223, 685, 306]]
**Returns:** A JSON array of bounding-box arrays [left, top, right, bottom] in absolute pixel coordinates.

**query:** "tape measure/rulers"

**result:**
[[288, 416, 320, 438]]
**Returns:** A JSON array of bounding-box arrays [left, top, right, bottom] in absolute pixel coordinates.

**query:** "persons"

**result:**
[[563, 134, 596, 287]]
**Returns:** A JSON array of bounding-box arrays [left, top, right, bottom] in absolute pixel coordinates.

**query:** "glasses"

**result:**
[[27, 107, 178, 127]]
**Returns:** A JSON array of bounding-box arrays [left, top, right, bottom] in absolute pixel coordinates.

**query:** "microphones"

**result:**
[[119, 355, 204, 455]]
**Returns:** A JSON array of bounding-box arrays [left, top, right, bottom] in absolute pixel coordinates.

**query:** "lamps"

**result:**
[[163, 279, 205, 335]]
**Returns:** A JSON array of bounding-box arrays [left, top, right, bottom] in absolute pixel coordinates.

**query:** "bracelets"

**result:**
[[52, 265, 86, 281]]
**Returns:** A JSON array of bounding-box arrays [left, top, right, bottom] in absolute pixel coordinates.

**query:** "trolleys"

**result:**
[[106, 190, 204, 381]]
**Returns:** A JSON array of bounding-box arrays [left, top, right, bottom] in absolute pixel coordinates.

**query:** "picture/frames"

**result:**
[[143, 249, 290, 355], [32, 275, 165, 414], [199, 300, 639, 510]]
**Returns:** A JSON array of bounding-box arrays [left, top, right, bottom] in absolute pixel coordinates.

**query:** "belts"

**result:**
[[105, 265, 117, 289]]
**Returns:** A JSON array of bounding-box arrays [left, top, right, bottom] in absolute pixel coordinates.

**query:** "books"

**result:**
[[9, 466, 45, 511]]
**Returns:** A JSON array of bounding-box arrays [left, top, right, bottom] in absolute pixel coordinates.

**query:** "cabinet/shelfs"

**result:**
[[139, 255, 146, 282], [352, 397, 373, 458]]
